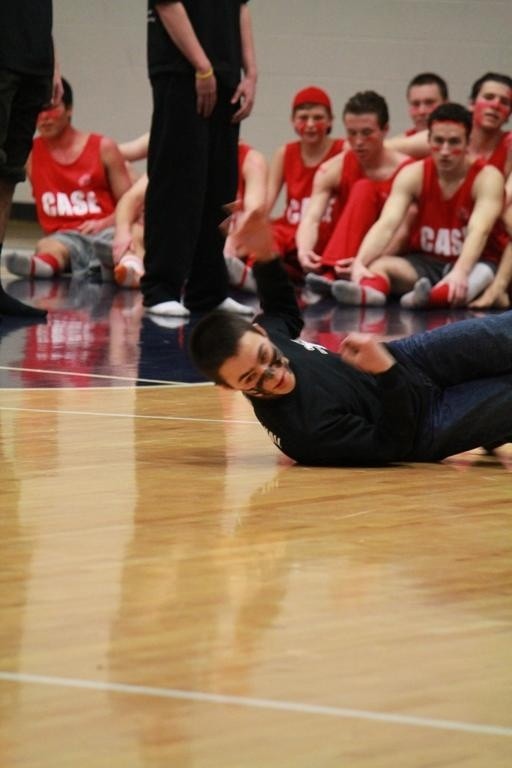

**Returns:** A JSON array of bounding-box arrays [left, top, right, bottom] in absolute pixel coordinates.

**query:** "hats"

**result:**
[[292, 85, 332, 118]]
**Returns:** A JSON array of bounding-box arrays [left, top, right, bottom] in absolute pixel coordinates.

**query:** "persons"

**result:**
[[141, 1, 263, 316], [2, 0, 63, 320], [197, 303, 511, 465], [0, 68, 512, 303]]
